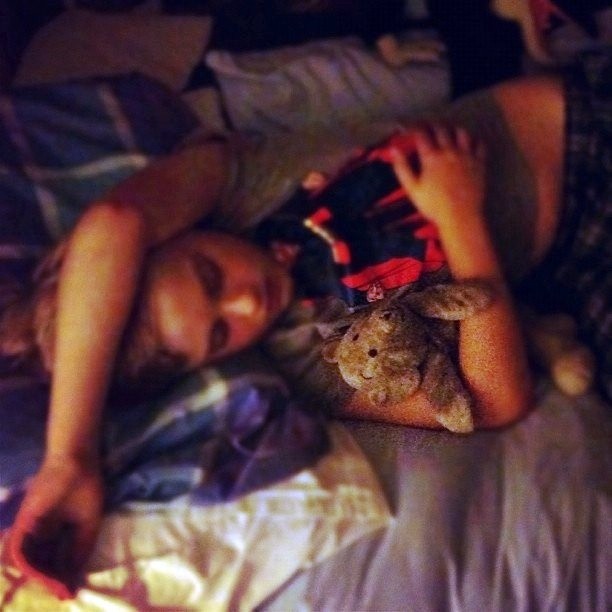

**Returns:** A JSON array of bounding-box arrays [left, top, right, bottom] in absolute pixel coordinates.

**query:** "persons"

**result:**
[[11, 74, 611, 598]]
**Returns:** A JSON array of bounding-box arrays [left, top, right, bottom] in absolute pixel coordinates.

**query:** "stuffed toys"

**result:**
[[321, 277, 596, 434]]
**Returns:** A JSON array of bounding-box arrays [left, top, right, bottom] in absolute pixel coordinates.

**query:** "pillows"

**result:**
[[0, 71, 326, 543], [202, 42, 455, 139]]
